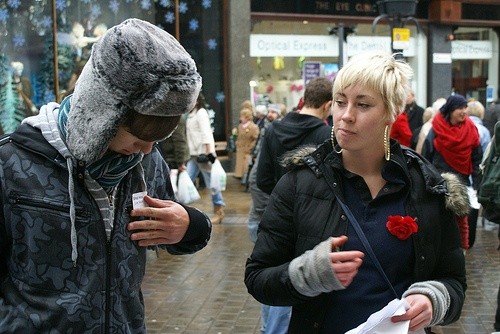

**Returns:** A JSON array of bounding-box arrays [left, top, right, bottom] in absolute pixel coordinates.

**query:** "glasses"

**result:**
[[119, 108, 180, 142]]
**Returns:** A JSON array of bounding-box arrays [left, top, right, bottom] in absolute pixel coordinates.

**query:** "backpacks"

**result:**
[[477, 122, 500, 207]]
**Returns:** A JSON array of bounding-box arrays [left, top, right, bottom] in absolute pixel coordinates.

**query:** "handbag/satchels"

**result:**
[[209, 159, 226, 192], [176, 169, 201, 205]]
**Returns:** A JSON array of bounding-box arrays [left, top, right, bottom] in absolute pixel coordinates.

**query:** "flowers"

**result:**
[[386, 215, 419, 240]]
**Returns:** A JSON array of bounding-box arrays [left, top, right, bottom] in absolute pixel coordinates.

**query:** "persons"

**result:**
[[257, 76, 335, 194], [158, 89, 286, 224], [485, 80, 493, 109], [246, 124, 293, 334], [1, 61, 37, 126], [244, 50, 468, 334], [67, 19, 107, 94], [388, 87, 500, 334], [0, 18, 213, 334]]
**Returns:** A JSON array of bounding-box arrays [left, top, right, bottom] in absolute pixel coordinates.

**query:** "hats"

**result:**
[[65, 18, 202, 160], [268, 104, 281, 116], [256, 105, 268, 116]]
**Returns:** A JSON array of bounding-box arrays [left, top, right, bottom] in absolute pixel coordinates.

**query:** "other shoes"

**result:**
[[209, 214, 224, 224]]
